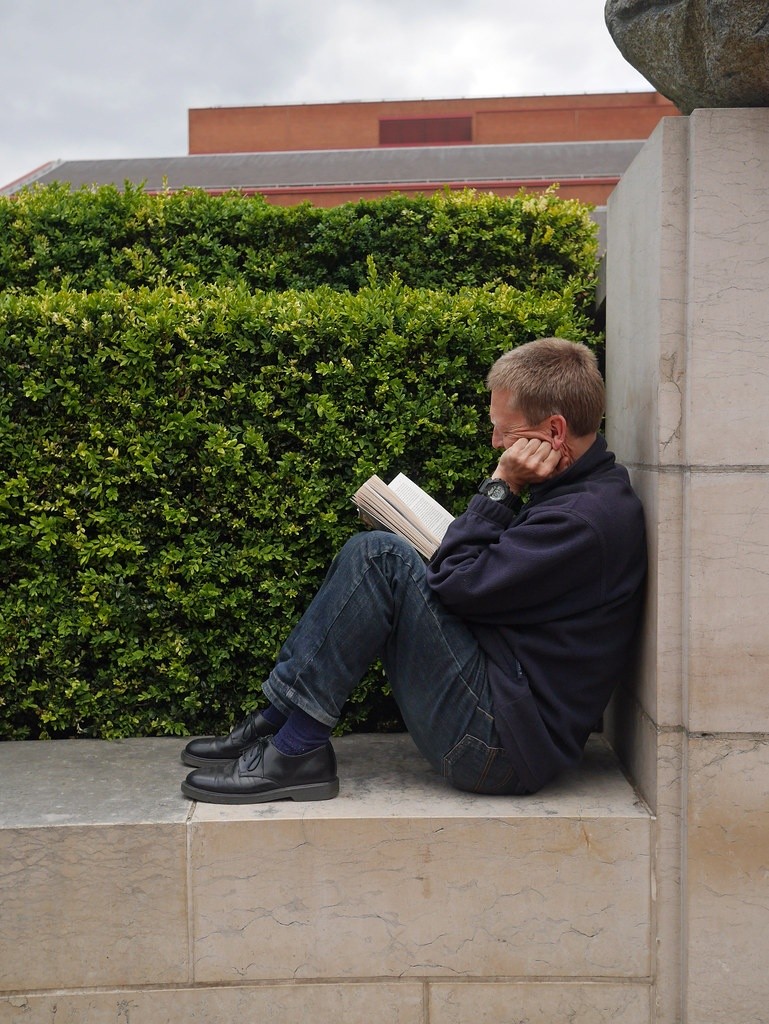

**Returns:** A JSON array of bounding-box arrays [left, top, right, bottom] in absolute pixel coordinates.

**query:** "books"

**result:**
[[350, 471, 458, 560]]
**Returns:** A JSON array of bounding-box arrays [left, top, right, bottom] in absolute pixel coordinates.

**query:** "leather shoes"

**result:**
[[181, 709, 339, 804]]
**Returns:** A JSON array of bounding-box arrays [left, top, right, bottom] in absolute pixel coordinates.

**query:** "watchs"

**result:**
[[478, 476, 524, 517]]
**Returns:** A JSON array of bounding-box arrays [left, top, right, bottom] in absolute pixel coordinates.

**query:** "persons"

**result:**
[[180, 338, 649, 804]]
